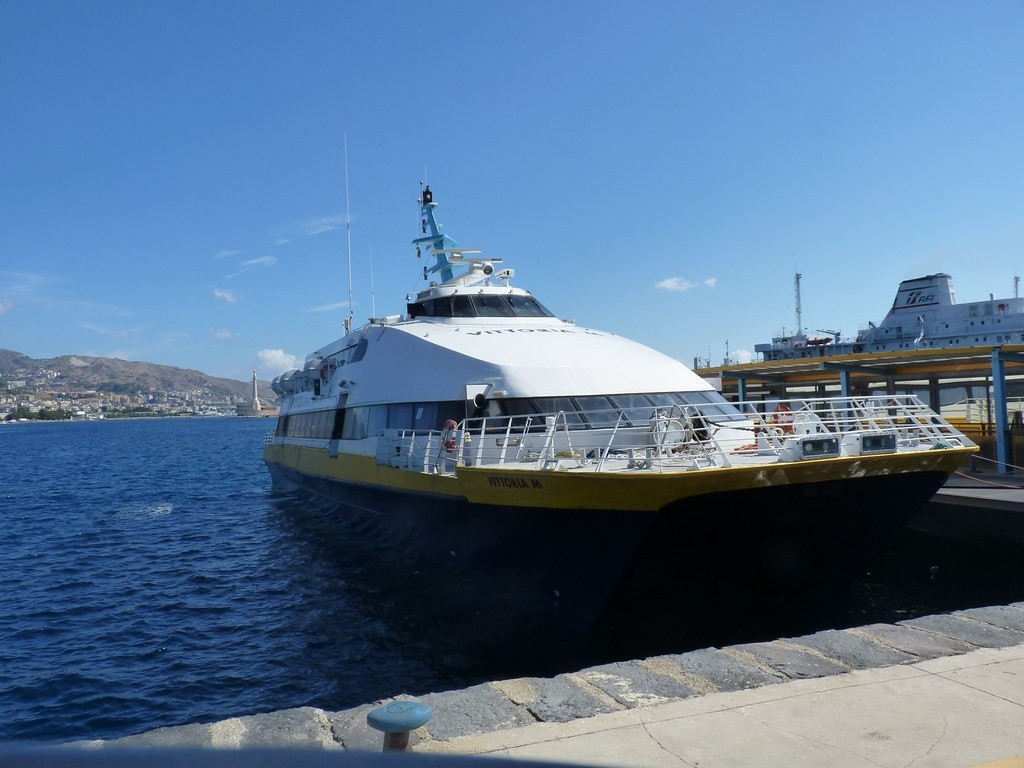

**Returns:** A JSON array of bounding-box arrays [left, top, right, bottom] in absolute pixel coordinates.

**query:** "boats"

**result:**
[[262, 159, 982, 602]]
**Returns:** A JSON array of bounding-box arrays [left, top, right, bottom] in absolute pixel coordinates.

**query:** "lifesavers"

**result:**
[[442, 419, 457, 453], [772, 405, 793, 433]]
